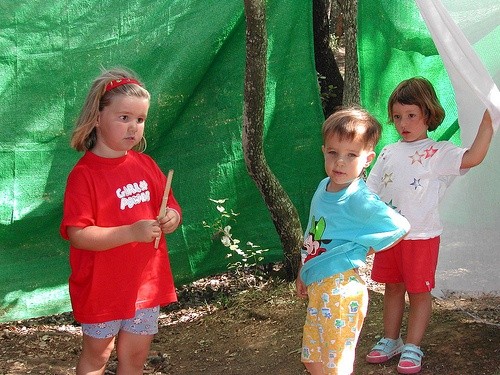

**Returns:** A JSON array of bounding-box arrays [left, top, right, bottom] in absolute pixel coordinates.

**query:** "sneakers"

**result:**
[[397, 343, 424, 373], [365, 336, 405, 364]]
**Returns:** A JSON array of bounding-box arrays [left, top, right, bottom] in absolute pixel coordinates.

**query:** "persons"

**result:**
[[363, 76, 500, 375], [59, 65, 182, 375], [294, 108, 411, 375]]
